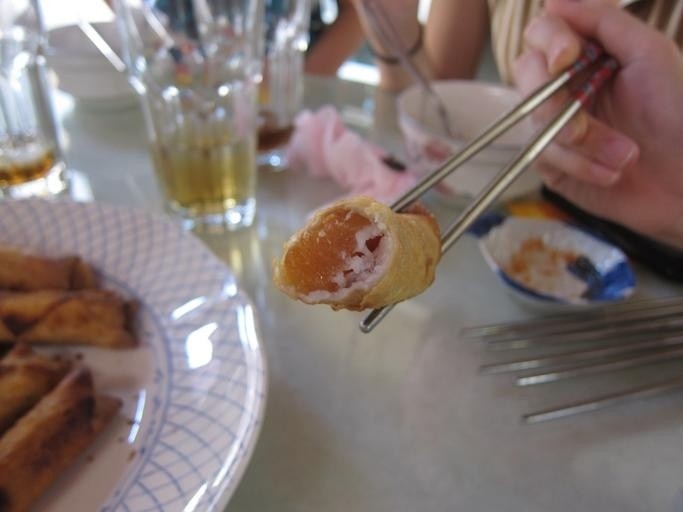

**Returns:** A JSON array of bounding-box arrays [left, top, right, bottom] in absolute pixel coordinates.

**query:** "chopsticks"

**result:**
[[459, 297, 682, 427], [327, 1, 642, 334], [460, 309, 641, 332]]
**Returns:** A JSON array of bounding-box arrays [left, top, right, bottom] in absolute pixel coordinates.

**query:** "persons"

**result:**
[[141, 0, 367, 78], [510, 1, 682, 251], [348, 0, 682, 90]]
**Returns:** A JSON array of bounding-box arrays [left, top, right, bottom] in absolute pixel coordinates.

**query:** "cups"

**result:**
[[115, 1, 257, 236], [0, 0, 69, 199], [192, 1, 307, 167]]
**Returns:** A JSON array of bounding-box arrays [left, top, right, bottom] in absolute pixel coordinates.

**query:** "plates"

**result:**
[[469, 212, 637, 309], [0, 197, 267, 512]]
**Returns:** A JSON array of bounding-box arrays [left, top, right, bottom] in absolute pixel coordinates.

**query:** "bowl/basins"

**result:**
[[395, 79, 543, 210]]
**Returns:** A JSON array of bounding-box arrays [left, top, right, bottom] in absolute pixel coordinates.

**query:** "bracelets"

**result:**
[[368, 21, 426, 65]]
